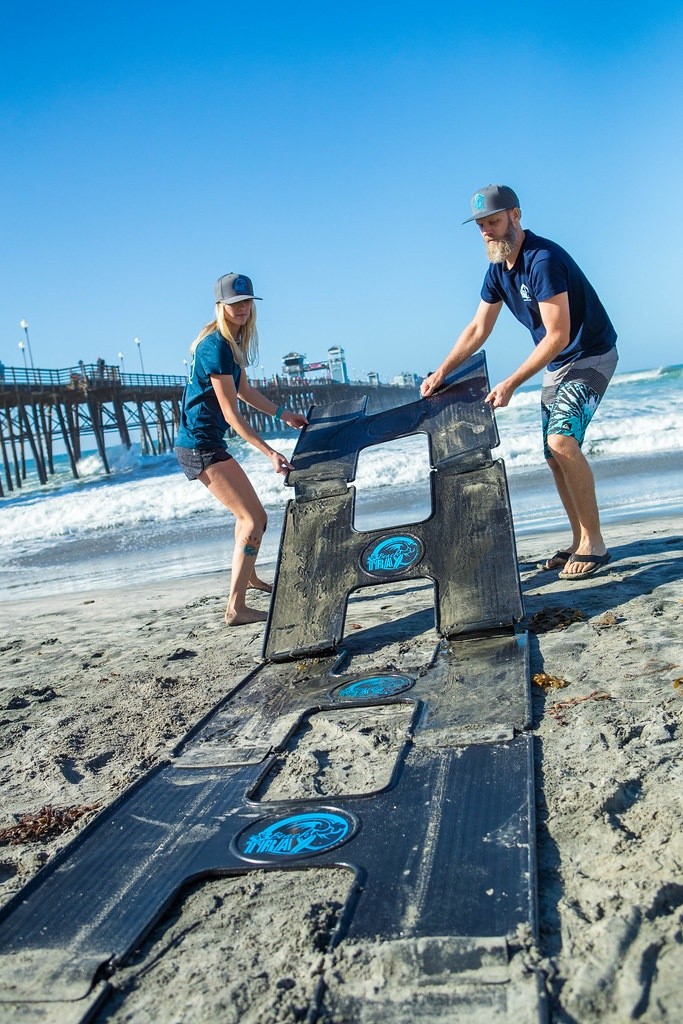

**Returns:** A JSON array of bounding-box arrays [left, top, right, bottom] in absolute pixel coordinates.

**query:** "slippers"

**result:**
[[537, 550, 611, 578]]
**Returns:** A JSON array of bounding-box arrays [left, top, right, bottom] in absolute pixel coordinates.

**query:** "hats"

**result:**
[[462, 184, 520, 225], [215, 272, 263, 304]]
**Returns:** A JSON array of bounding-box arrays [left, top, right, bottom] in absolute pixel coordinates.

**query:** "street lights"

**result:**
[[119, 352, 125, 373], [20, 318, 37, 383], [135, 337, 144, 373], [183, 359, 188, 375], [19, 341, 30, 385]]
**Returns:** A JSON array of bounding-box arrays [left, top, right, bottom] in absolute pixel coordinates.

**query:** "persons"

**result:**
[[173, 271, 312, 626], [418, 184, 621, 579]]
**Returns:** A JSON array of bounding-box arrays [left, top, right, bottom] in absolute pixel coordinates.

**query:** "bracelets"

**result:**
[[273, 404, 285, 421]]
